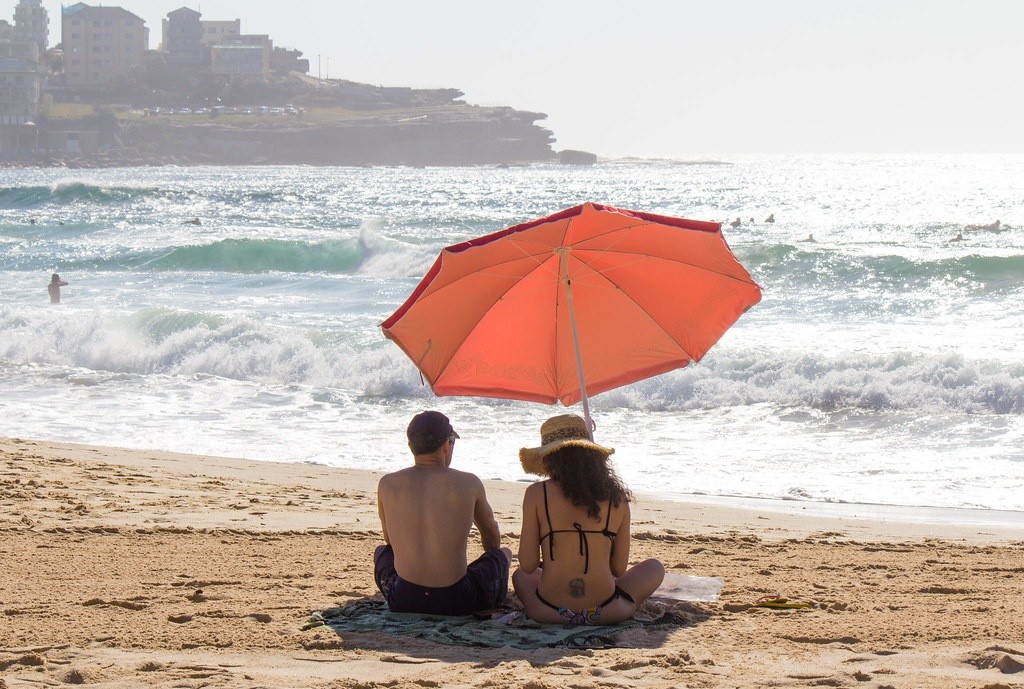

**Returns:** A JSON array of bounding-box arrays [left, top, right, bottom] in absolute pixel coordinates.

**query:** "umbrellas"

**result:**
[[377, 201, 765, 444]]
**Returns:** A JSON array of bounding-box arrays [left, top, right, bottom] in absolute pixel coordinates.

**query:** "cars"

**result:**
[[143, 102, 298, 116]]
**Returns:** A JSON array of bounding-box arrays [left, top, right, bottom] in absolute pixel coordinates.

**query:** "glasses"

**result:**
[[438, 436, 455, 444]]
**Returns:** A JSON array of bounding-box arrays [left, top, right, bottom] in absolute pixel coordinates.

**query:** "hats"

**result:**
[[407, 410, 460, 439], [519, 414, 614, 476]]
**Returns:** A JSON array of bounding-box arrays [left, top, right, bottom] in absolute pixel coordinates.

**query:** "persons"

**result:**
[[947, 220, 1003, 242], [181, 217, 201, 226], [373, 411, 512, 616], [798, 234, 818, 243], [681, 214, 775, 225], [47, 273, 69, 304], [512, 413, 665, 625], [30, 218, 35, 224], [60, 222, 65, 225]]
